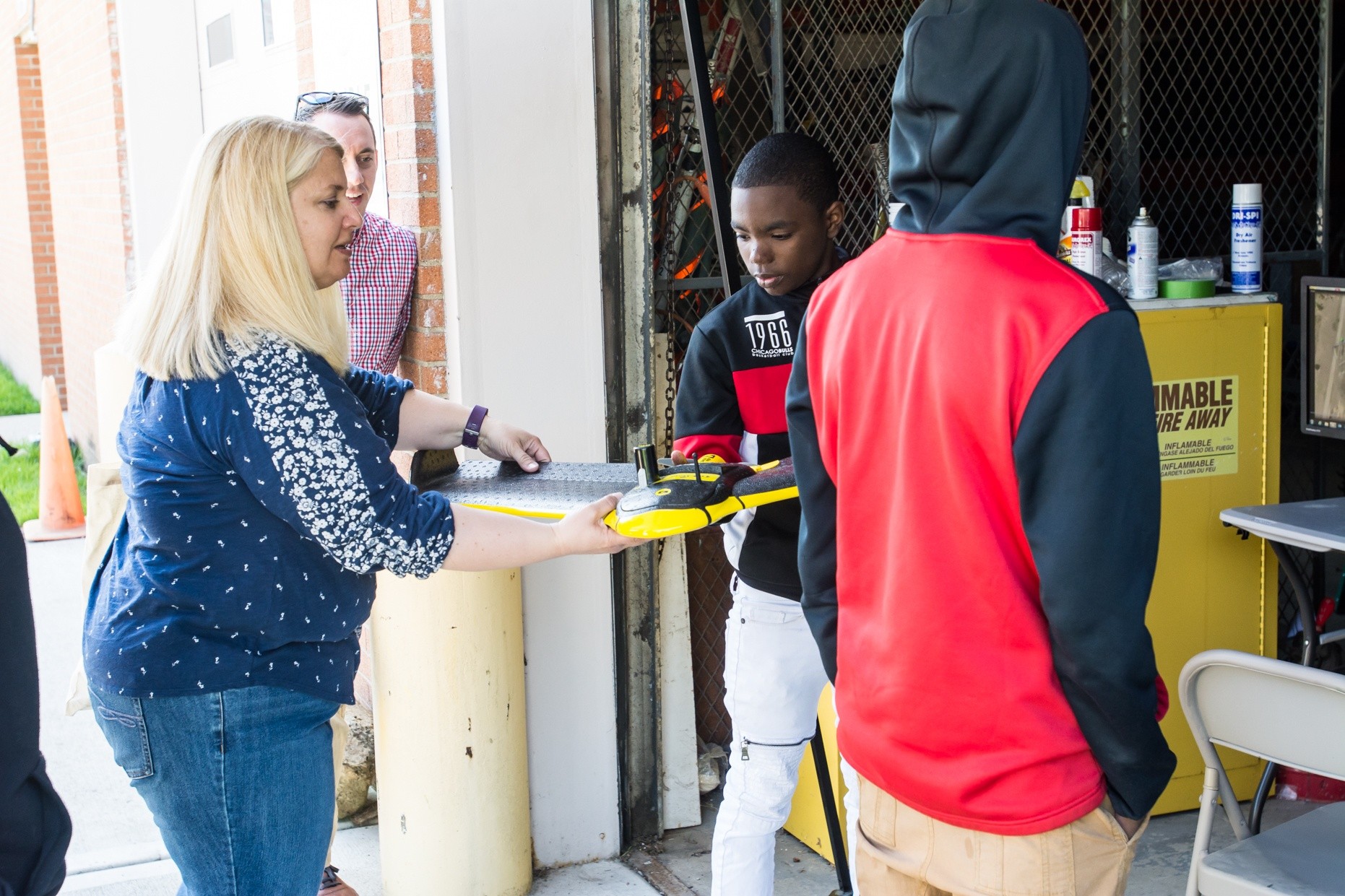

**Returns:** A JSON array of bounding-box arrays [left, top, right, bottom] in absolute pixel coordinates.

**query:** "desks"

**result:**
[[1218, 496, 1344, 836]]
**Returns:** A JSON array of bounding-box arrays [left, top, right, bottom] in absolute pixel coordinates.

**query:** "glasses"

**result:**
[[293, 92, 370, 121]]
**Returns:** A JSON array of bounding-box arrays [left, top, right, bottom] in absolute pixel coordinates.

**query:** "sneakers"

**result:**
[[318, 865, 360, 896]]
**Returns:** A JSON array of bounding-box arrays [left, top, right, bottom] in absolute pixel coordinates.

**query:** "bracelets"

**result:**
[[461, 405, 489, 450]]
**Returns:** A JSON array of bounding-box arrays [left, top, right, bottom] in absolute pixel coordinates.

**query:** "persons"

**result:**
[[80, 94, 651, 896], [670, 129, 856, 896], [784, 0, 1178, 896], [288, 93, 417, 823]]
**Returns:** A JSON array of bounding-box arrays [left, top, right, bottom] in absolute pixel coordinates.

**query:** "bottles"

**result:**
[[1232, 184, 1264, 293], [1072, 208, 1102, 281], [1102, 252, 1132, 297], [1126, 207, 1159, 299]]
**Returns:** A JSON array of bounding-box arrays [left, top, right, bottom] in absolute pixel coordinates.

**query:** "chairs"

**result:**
[[1178, 647, 1344, 896]]
